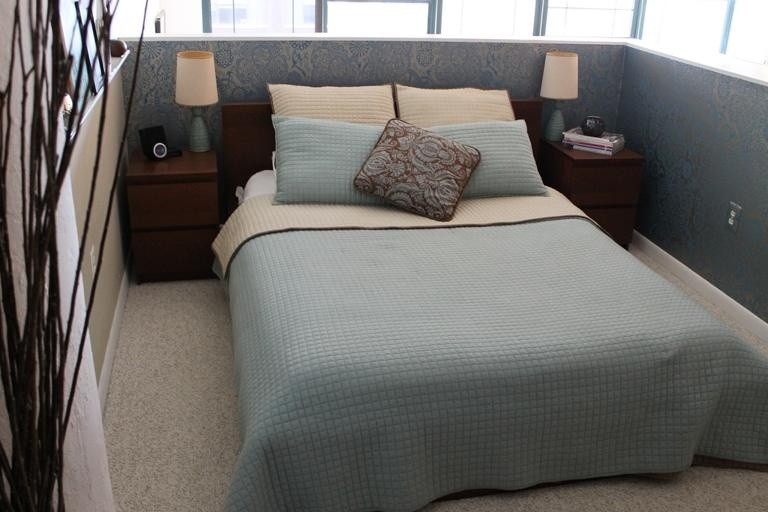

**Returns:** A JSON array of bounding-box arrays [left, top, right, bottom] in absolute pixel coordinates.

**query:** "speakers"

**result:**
[[138, 125, 169, 161]]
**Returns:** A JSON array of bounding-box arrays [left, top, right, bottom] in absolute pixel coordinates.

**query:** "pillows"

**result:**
[[355, 117, 481, 222], [421, 118, 546, 196], [266, 83, 396, 123], [393, 83, 516, 128], [271, 113, 389, 208]]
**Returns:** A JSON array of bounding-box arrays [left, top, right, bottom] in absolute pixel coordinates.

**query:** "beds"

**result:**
[[219, 101, 732, 511]]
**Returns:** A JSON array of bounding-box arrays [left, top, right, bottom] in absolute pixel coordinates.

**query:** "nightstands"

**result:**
[[124, 144, 221, 284], [539, 136, 642, 251]]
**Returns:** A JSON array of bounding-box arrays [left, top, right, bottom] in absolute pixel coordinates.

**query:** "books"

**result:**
[[560, 126, 626, 157]]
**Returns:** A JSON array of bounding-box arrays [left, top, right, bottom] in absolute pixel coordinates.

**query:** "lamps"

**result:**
[[538, 51, 578, 141], [175, 50, 218, 153]]
